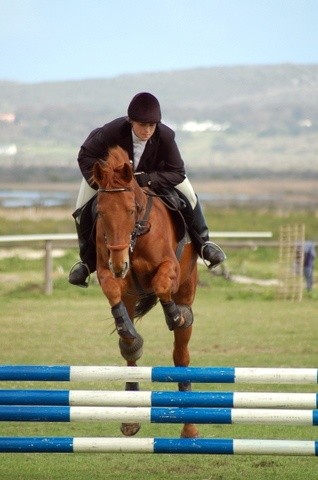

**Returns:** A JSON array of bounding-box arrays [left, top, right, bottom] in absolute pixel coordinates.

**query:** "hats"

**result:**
[[127, 92, 162, 124]]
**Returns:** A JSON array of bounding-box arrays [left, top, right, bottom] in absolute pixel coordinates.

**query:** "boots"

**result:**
[[69, 202, 99, 283], [179, 193, 225, 264]]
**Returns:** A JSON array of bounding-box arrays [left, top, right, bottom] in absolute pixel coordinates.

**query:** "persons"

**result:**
[[296, 239, 315, 291], [68, 92, 227, 288]]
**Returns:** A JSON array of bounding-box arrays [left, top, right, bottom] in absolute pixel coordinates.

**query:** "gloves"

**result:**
[[136, 173, 151, 187]]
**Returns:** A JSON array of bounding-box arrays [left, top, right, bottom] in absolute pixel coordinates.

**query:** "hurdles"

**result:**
[[0, 365, 318, 457]]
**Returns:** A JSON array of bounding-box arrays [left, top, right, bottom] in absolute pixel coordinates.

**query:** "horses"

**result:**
[[95, 147, 199, 438]]
